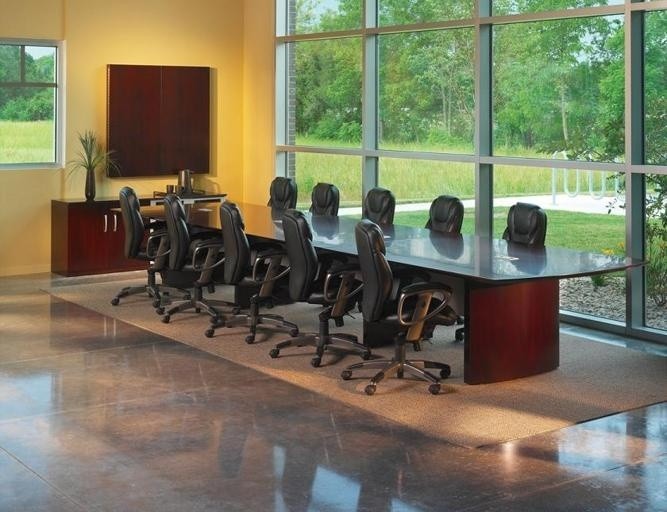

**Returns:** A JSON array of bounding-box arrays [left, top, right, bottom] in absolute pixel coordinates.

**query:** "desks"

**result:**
[[110, 199, 649, 384]]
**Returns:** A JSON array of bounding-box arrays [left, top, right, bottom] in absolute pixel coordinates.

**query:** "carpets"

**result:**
[[40, 275, 666, 450]]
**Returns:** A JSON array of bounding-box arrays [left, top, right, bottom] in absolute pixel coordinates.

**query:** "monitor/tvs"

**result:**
[[106, 63, 211, 178]]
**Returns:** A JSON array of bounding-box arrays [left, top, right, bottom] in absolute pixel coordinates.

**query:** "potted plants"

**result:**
[[56, 129, 124, 200]]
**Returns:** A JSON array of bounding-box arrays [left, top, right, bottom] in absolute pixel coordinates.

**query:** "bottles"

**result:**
[[178, 169, 192, 194]]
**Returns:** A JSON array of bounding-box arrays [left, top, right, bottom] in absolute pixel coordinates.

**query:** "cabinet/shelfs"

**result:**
[[50, 198, 221, 276]]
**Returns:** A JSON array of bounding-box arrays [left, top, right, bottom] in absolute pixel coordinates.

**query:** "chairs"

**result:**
[[119, 177, 547, 396]]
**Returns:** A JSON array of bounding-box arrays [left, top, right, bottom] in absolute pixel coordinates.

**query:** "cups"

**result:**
[[166, 185, 183, 194]]
